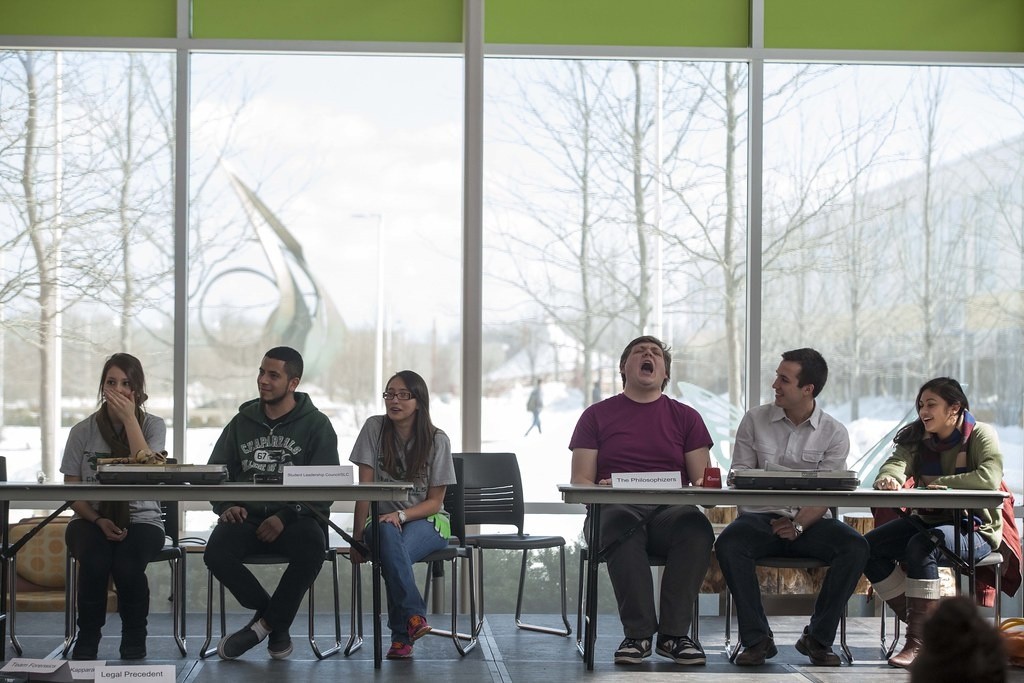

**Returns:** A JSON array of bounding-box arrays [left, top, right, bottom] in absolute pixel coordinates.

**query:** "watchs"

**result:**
[[791, 521, 803, 535], [398, 509, 407, 524]]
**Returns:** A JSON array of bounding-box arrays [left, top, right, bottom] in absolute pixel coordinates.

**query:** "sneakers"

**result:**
[[613, 636, 653, 665], [267, 631, 293, 660], [655, 633, 706, 666], [218, 618, 267, 660], [386, 641, 414, 658], [407, 616, 432, 642]]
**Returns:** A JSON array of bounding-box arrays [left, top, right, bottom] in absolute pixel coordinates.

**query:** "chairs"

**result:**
[[0, 453, 1005, 665]]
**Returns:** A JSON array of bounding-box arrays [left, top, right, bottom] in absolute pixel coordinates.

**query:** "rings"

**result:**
[[881, 482, 886, 486]]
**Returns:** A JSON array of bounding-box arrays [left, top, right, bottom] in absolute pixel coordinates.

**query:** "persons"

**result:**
[[569, 335, 714, 664], [593, 383, 601, 402], [907, 593, 1008, 683], [202, 346, 340, 660], [348, 370, 457, 658], [524, 378, 545, 437], [861, 376, 1002, 666], [717, 348, 868, 665], [59, 353, 167, 660]]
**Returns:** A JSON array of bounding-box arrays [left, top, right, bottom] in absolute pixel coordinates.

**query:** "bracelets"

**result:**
[[95, 516, 103, 524]]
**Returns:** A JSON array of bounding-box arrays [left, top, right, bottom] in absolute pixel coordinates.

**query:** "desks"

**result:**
[[0, 481, 414, 671], [556, 484, 1010, 671]]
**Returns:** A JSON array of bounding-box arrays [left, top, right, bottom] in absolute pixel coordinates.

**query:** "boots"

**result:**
[[888, 577, 941, 668], [870, 565, 908, 624]]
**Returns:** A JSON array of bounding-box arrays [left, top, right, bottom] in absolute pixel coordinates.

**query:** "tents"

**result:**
[[491, 321, 616, 381]]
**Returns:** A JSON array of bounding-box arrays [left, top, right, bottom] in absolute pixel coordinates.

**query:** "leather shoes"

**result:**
[[795, 625, 841, 666], [735, 638, 779, 665]]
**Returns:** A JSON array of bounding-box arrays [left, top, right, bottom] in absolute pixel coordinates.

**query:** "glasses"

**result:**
[[382, 391, 416, 400]]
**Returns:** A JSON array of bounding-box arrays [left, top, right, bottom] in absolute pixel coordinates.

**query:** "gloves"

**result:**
[[427, 513, 450, 539]]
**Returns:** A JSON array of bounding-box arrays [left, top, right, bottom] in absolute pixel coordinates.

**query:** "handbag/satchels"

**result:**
[[997, 618, 1024, 667]]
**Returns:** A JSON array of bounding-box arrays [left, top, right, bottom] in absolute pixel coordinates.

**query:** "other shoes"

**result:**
[[119, 623, 148, 661], [71, 629, 102, 660]]
